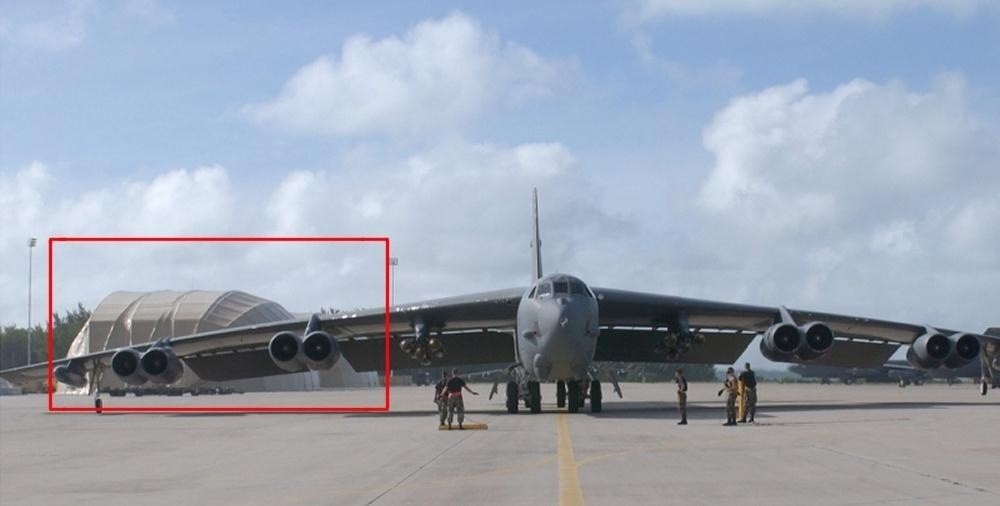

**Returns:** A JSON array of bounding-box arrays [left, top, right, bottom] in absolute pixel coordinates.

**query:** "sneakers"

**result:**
[[678, 416, 755, 426], [441, 419, 464, 430]]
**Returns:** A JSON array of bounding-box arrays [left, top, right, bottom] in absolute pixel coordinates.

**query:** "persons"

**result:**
[[673, 367, 689, 426], [739, 362, 758, 423], [721, 367, 738, 426], [441, 368, 481, 431], [434, 371, 450, 427]]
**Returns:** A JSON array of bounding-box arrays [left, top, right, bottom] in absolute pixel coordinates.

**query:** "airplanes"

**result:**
[[0, 188, 1000, 413]]
